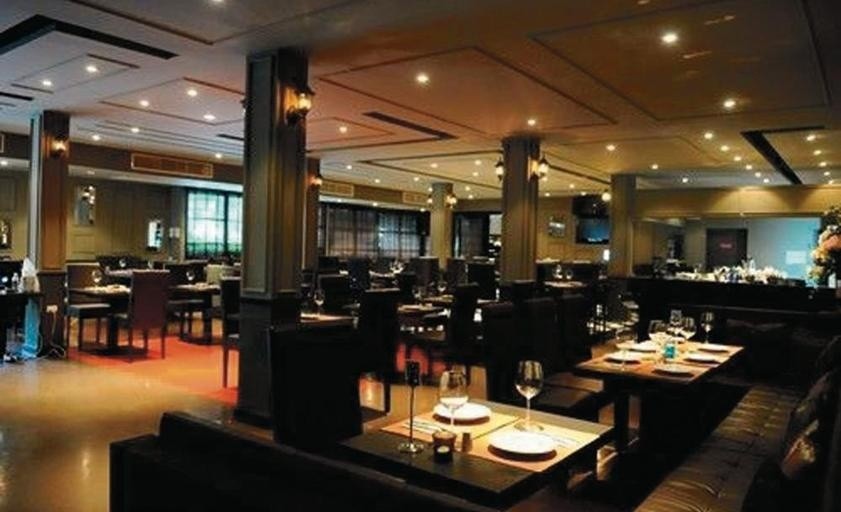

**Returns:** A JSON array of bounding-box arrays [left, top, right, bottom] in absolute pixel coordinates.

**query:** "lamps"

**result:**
[[446, 194, 458, 209], [310, 174, 324, 192], [287, 76, 316, 127], [530, 153, 550, 182], [495, 156, 505, 182], [52, 132, 69, 159]]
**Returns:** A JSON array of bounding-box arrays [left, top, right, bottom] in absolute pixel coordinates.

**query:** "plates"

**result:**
[[489, 431, 556, 455], [699, 343, 728, 353], [604, 351, 641, 361], [629, 343, 655, 351], [653, 363, 688, 374], [433, 402, 490, 423], [685, 353, 715, 362]]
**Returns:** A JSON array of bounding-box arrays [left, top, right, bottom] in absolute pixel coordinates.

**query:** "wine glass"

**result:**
[[439, 370, 469, 435], [313, 290, 324, 317], [700, 312, 714, 344], [146, 257, 154, 270], [90, 268, 102, 290], [515, 359, 543, 430], [186, 270, 195, 287], [437, 280, 446, 298], [617, 329, 634, 370], [118, 257, 127, 271], [411, 285, 422, 308], [649, 312, 696, 363], [566, 270, 573, 286]]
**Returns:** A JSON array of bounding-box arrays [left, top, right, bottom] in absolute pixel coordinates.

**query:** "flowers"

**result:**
[[809, 210, 841, 291]]
